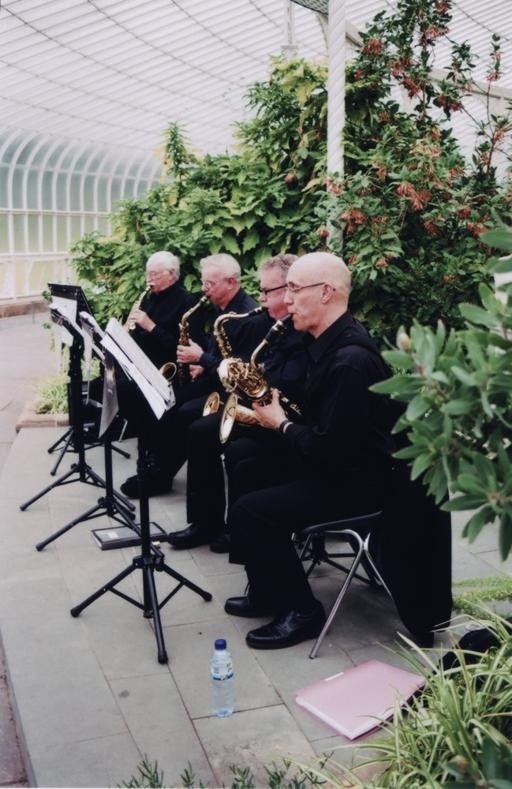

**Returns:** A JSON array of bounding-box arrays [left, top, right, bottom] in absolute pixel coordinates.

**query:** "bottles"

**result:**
[[210, 638, 236, 717]]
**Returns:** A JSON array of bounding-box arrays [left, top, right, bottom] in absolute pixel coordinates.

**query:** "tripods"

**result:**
[[19, 338, 136, 511], [35, 364, 166, 559], [47, 338, 131, 475], [70, 393, 213, 664]]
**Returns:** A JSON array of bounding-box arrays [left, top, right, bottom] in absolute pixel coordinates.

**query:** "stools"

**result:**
[[295, 507, 392, 659]]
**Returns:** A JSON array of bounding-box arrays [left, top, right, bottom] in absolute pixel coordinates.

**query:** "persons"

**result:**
[[119, 252, 274, 498], [164, 252, 313, 555], [82, 249, 200, 445], [223, 251, 416, 650]]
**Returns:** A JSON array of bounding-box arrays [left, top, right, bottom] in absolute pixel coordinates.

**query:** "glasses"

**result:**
[[258, 284, 287, 295], [284, 283, 336, 296]]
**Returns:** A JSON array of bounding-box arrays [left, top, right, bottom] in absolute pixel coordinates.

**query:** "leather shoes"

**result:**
[[120, 473, 327, 648]]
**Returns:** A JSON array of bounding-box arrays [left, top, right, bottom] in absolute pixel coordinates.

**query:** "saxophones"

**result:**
[[159, 296, 210, 384], [202, 306, 267, 416], [217, 314, 305, 445]]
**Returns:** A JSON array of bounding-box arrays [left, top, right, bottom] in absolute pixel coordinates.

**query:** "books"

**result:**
[[90, 521, 167, 551], [292, 656, 429, 740]]
